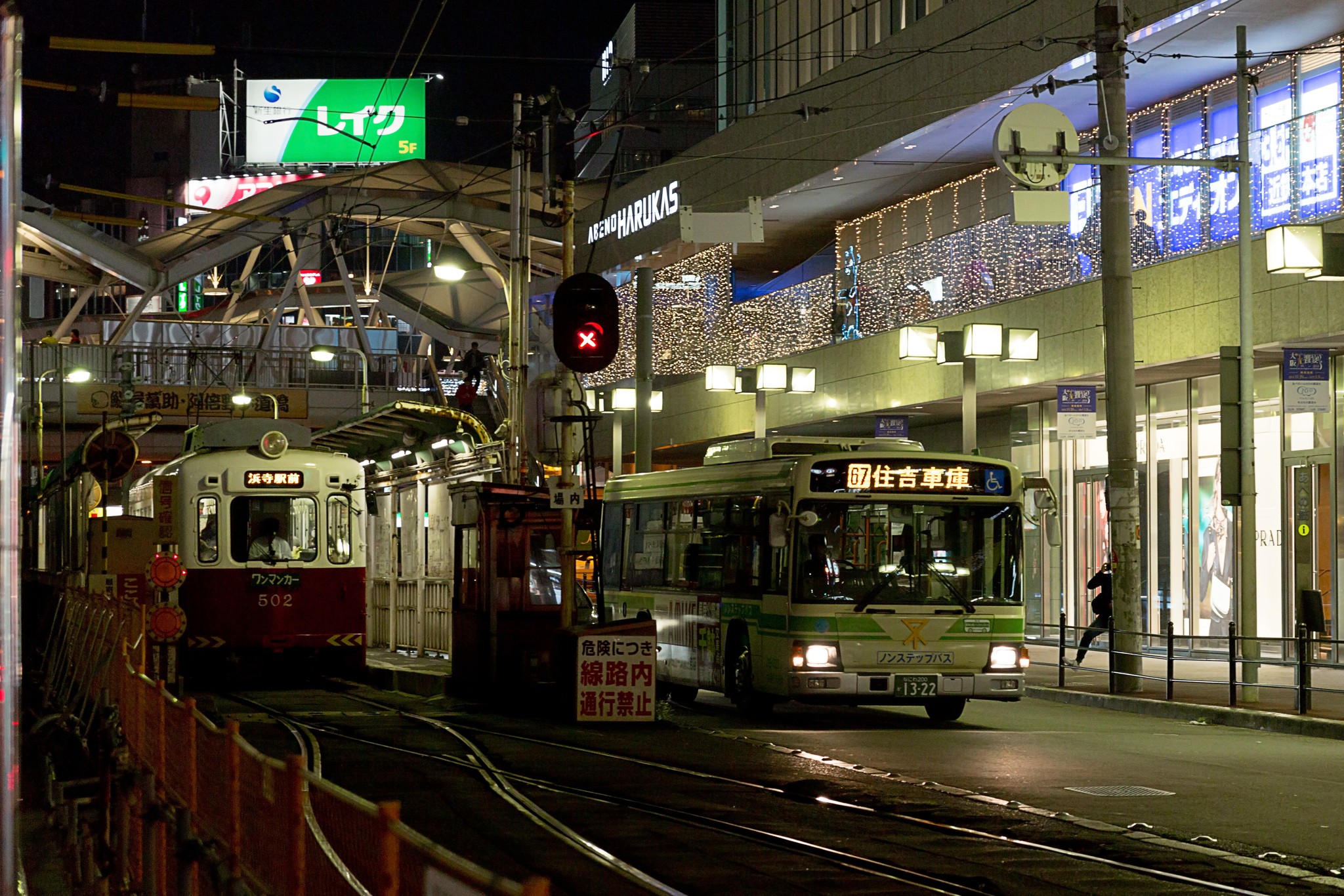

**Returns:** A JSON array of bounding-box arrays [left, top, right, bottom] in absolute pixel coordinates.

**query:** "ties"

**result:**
[[268, 539, 274, 555]]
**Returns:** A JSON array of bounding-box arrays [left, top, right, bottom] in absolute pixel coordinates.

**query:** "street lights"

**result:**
[[581, 388, 664, 477], [899, 322, 1041, 458], [595, 436, 1067, 723], [231, 389, 280, 420], [308, 321, 372, 415], [433, 89, 525, 482], [705, 363, 816, 440], [36, 361, 91, 479], [596, 225, 676, 284]]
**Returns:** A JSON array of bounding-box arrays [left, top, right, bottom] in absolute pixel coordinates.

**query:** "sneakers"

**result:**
[[1062, 657, 1079, 672]]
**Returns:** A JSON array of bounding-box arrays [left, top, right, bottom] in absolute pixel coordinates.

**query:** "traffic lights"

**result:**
[[554, 272, 620, 373]]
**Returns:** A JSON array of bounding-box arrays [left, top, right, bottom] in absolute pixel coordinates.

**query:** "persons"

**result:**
[[1200, 459, 1235, 637], [247, 517, 293, 560], [799, 533, 847, 599], [461, 341, 486, 395], [1061, 552, 1113, 672], [455, 376, 475, 415], [1097, 481, 1110, 560], [200, 515, 217, 541]]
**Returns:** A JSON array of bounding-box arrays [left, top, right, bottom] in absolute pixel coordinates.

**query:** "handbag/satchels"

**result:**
[[1091, 593, 1112, 615], [1210, 574, 1231, 618]]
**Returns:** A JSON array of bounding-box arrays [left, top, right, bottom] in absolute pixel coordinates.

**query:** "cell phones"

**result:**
[[1106, 562, 1112, 571]]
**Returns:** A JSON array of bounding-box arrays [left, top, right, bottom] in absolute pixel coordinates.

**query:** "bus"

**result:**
[[4, 435, 397, 724]]
